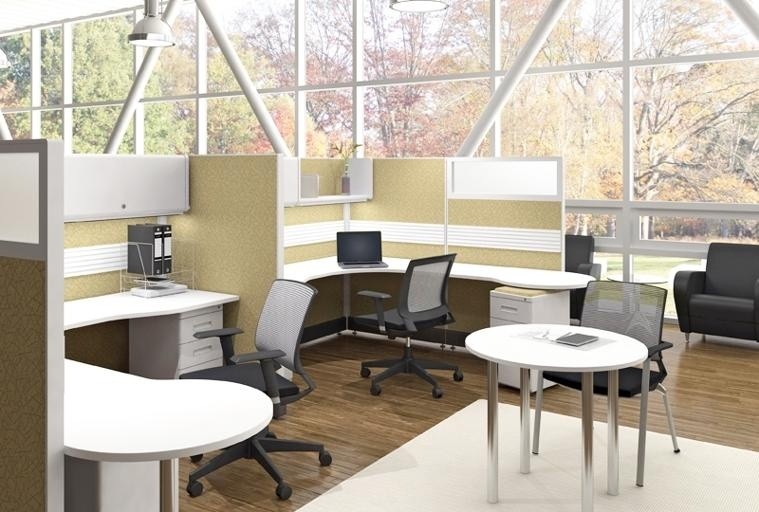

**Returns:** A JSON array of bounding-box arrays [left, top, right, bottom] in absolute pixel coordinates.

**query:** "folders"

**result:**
[[128, 224, 162, 275], [145, 224, 173, 274]]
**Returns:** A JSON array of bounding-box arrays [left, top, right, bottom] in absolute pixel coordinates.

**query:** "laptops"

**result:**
[[336, 230, 388, 269]]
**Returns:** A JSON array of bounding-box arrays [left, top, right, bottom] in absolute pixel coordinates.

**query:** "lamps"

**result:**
[[389, 0, 449, 16], [127, 0, 176, 49]]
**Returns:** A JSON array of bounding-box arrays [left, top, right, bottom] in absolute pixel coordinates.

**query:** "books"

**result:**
[[556, 334, 598, 347]]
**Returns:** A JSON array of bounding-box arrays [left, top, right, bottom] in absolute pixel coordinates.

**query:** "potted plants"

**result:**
[[329, 140, 363, 196]]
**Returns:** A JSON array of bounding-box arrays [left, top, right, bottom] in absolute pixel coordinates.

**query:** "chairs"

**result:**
[[178, 279, 332, 500], [352, 253, 463, 401], [565, 235, 602, 325], [531, 279, 681, 489], [673, 242, 759, 349]]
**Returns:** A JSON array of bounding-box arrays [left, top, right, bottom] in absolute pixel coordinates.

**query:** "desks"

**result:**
[[64, 283, 274, 511], [464, 324, 649, 512], [283, 255, 597, 395]]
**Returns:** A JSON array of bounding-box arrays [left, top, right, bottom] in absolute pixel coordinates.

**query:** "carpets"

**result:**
[[295, 399, 759, 512]]
